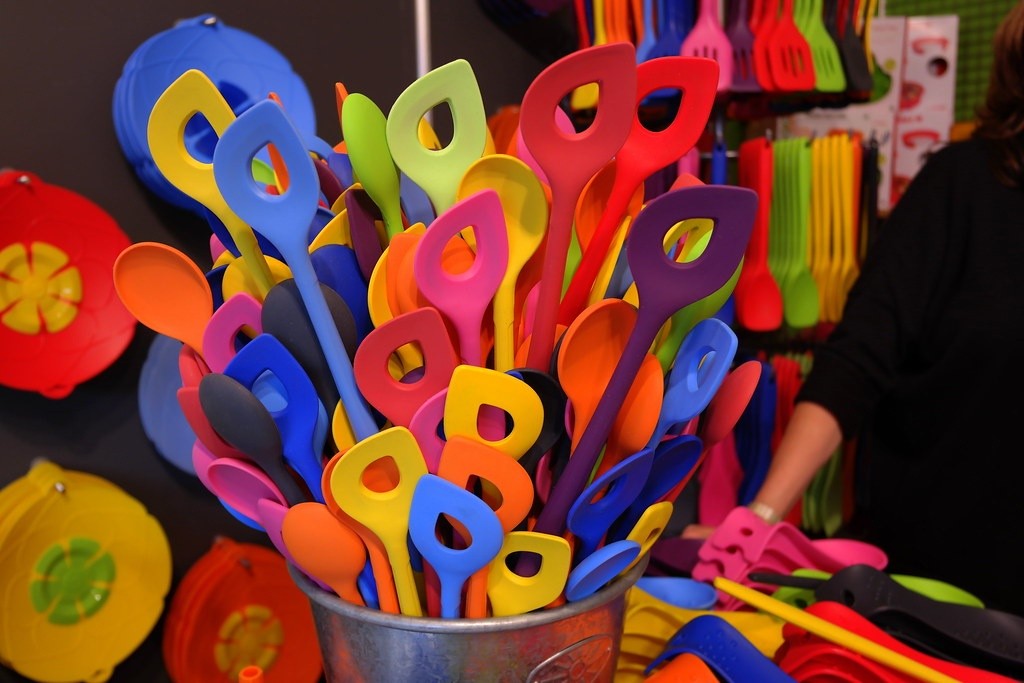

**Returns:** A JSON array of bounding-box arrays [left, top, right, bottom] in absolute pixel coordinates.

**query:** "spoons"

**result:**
[[635, 574, 719, 609], [690, 507, 987, 610], [506, 297, 666, 493], [110, 241, 212, 348], [652, 220, 745, 371], [668, 361, 767, 500], [457, 154, 547, 373], [735, 132, 863, 330], [802, 439, 858, 535], [339, 91, 403, 244], [573, 157, 646, 313], [169, 276, 374, 608], [565, 541, 643, 602]]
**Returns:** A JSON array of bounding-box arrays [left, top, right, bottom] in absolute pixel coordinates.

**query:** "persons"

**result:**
[[679, 0, 1024, 617]]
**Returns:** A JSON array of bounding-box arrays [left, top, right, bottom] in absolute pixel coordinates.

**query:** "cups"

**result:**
[[271, 550, 653, 683]]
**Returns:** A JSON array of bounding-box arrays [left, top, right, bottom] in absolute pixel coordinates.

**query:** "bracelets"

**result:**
[[749, 500, 781, 526]]
